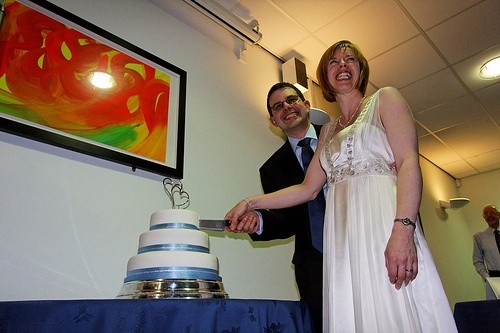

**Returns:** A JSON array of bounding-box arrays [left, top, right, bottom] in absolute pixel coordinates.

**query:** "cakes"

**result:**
[[124, 177, 223, 283]]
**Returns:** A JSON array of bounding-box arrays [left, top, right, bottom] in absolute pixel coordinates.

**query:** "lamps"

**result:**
[[439, 198, 470, 220], [184, 0, 262, 66]]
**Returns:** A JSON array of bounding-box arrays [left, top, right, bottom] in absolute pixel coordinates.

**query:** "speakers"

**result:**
[[282, 57, 308, 94]]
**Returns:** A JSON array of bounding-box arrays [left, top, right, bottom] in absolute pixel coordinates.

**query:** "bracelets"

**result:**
[[394, 218, 417, 228], [243, 198, 253, 211]]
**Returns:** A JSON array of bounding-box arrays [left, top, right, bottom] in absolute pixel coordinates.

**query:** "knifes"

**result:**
[[198, 218, 257, 231]]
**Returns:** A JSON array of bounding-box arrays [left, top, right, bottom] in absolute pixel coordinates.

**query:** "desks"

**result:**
[[0, 298, 310, 333], [453, 299, 500, 333]]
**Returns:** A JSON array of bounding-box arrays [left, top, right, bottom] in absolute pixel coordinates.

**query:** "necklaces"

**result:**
[[339, 95, 366, 128]]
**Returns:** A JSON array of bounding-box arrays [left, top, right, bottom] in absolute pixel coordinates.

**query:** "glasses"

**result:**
[[270, 95, 299, 112]]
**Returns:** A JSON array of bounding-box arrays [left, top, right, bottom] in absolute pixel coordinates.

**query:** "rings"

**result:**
[[406, 269, 413, 272]]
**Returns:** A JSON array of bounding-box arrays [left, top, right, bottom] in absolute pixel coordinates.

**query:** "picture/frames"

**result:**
[[0, 0, 187, 180]]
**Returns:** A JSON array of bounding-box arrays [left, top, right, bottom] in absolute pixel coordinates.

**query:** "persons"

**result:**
[[224, 40, 461, 333], [472, 203, 500, 282], [235, 81, 323, 333]]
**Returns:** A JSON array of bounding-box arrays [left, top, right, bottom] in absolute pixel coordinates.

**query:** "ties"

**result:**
[[297, 137, 326, 253], [494, 230, 500, 254]]
